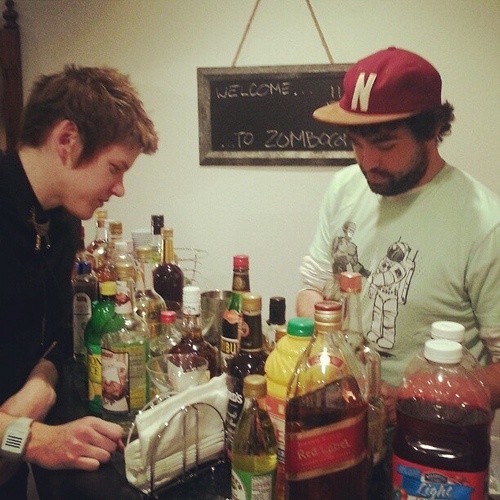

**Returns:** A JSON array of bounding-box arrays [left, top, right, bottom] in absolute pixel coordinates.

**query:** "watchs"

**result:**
[[1, 415, 33, 457]]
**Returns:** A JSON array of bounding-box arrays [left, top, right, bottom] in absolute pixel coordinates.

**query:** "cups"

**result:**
[[145, 353, 208, 393]]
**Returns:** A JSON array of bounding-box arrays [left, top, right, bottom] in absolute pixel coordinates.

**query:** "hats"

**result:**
[[312, 47, 443, 125]]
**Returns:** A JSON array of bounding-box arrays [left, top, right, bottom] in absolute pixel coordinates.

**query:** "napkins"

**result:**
[[124, 373, 229, 492]]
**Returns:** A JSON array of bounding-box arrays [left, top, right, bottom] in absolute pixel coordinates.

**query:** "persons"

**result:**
[[0, 63, 158, 500], [296, 47, 500, 404]]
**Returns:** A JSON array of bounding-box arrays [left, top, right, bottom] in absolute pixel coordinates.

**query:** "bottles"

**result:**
[[70, 211, 496, 500]]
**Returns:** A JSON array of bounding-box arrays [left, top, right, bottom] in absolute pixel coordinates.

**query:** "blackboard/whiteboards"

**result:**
[[196, 63, 358, 167]]
